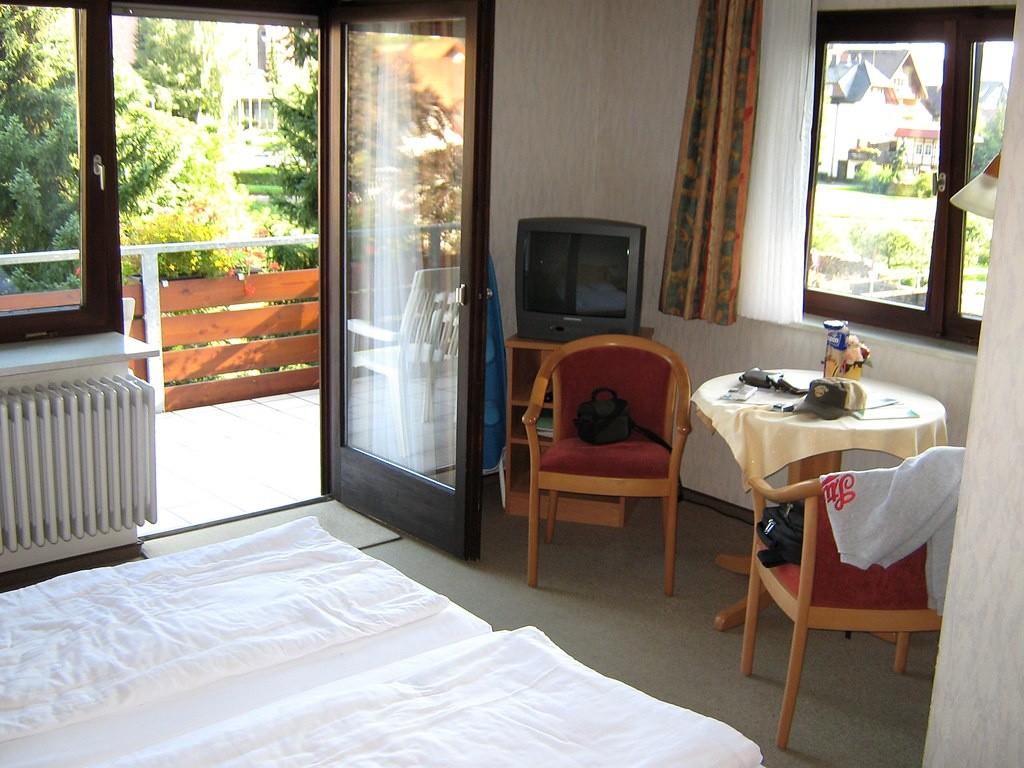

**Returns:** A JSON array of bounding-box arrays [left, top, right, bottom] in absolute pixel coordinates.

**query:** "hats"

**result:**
[[794, 376, 866, 420]]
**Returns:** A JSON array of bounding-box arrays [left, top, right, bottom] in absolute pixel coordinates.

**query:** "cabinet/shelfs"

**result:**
[[504, 325, 657, 529]]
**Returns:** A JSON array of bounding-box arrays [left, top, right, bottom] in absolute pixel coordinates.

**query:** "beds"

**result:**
[[0, 515, 769, 768]]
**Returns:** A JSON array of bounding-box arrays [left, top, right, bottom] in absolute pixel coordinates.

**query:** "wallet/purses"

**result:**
[[738, 366, 784, 389]]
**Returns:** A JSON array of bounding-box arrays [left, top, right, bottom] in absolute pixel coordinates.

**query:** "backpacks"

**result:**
[[756, 502, 804, 568]]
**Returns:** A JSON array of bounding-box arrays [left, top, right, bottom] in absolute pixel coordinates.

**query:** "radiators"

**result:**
[[0, 373, 158, 557]]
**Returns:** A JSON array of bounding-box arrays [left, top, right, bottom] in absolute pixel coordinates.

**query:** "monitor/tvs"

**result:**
[[515, 216, 646, 343]]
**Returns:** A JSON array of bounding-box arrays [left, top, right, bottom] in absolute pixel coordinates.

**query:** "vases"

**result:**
[[839, 363, 863, 381]]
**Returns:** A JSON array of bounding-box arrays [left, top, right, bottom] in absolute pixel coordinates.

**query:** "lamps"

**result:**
[[949, 146, 1002, 220]]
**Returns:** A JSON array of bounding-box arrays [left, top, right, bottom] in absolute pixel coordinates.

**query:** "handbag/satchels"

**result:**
[[574, 386, 630, 444]]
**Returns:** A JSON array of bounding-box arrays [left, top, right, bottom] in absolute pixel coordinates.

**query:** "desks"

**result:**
[[688, 366, 949, 647]]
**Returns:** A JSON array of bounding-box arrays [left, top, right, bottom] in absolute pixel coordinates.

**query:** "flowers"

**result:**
[[842, 334, 875, 365]]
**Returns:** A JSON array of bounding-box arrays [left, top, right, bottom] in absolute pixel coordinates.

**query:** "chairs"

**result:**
[[346, 267, 461, 459], [739, 446, 973, 750], [521, 333, 693, 598]]
